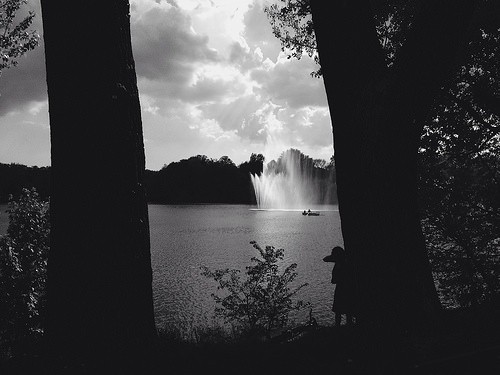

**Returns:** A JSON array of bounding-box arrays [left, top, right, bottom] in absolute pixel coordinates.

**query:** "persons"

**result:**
[[322, 246, 357, 326]]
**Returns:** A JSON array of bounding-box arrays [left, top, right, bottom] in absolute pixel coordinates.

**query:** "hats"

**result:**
[[323, 246, 353, 262]]
